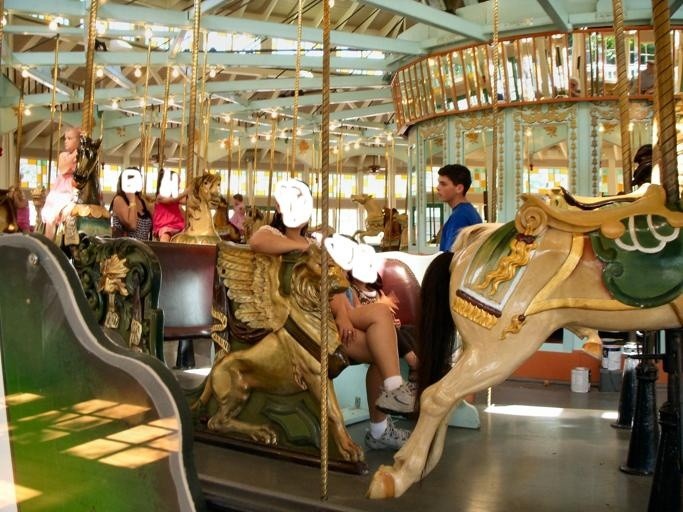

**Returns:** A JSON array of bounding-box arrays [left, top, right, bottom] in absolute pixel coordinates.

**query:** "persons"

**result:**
[[108, 167, 153, 242], [227, 194, 246, 230], [411, 161, 483, 421], [41, 128, 86, 241], [13, 187, 30, 232], [152, 171, 194, 243], [348, 270, 419, 384], [256, 180, 418, 449]]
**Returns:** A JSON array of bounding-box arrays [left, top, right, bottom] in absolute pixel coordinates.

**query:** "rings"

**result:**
[[348, 330, 352, 333]]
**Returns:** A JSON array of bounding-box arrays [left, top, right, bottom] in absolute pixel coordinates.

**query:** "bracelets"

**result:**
[[129, 201, 135, 207]]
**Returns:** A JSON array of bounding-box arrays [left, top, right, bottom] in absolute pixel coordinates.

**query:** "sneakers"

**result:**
[[364, 424, 411, 452], [374, 384, 417, 414], [408, 367, 417, 379]]
